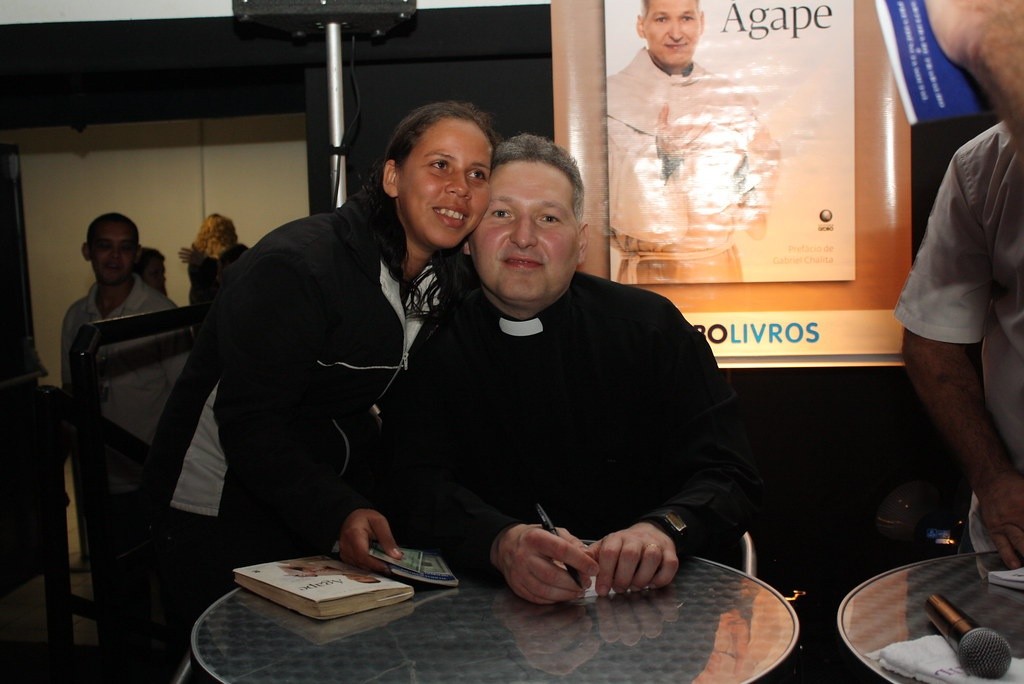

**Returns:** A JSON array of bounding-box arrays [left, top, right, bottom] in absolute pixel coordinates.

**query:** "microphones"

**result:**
[[924, 592, 1013, 680]]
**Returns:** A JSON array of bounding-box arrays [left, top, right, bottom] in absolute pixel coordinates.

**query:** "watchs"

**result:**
[[645, 509, 692, 544]]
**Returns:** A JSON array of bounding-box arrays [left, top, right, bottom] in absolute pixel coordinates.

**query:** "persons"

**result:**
[[61, 213, 191, 610], [130, 100, 497, 629], [135, 246, 167, 294], [179, 244, 255, 335], [892, 118, 1024, 568], [603, 0, 785, 286], [381, 132, 766, 607], [193, 214, 238, 259]]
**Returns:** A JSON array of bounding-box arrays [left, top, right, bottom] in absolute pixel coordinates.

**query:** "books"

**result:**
[[987, 567, 1024, 589], [233, 554, 415, 620], [390, 550, 460, 587]]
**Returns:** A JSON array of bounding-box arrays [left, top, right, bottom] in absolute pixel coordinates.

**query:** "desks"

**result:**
[[188, 538, 802, 684], [836, 547, 1024, 684]]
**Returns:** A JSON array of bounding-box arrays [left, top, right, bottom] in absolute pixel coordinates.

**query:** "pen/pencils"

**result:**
[[534, 500, 584, 590]]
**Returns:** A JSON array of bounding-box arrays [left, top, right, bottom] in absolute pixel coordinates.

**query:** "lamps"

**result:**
[[874, 479, 958, 545]]
